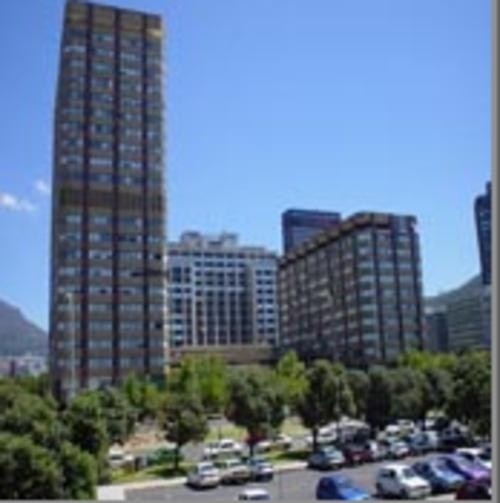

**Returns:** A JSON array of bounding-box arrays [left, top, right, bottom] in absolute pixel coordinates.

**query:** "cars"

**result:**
[[437, 454, 491, 489], [373, 463, 432, 501], [237, 486, 273, 501], [455, 447, 491, 471], [316, 472, 372, 502], [476, 443, 492, 456], [410, 457, 465, 496]]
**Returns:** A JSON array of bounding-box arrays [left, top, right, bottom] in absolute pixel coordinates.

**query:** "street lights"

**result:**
[[64, 292, 77, 402]]
